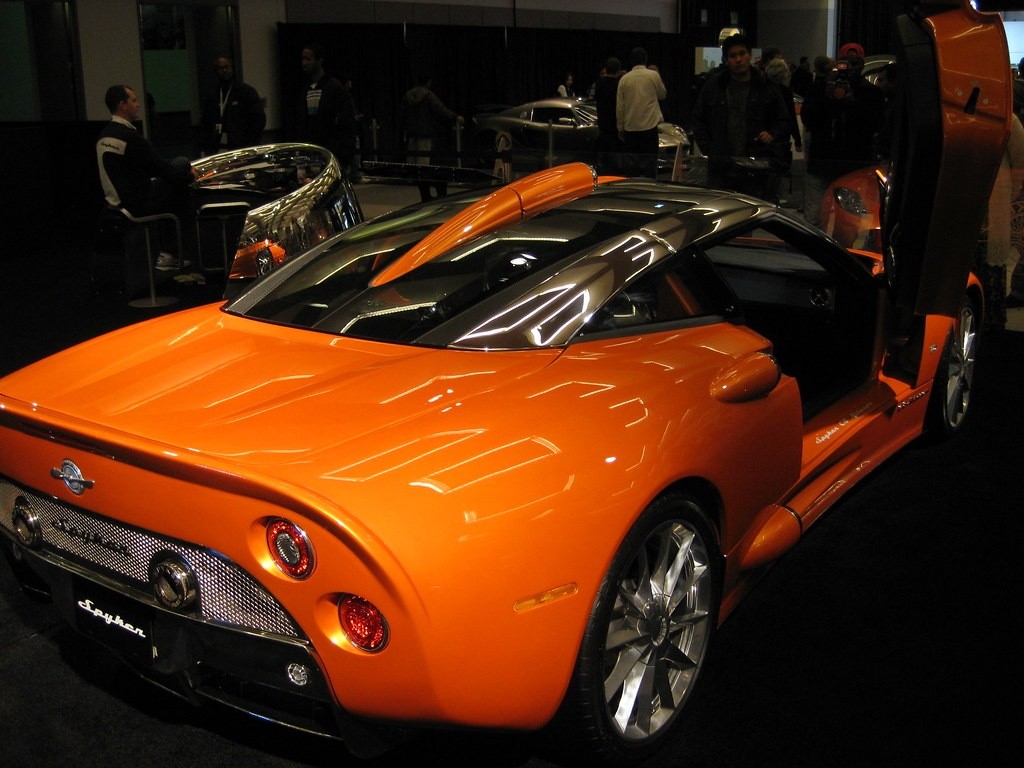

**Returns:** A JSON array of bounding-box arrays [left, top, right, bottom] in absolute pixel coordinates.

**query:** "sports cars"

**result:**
[[1, 0, 1012, 768]]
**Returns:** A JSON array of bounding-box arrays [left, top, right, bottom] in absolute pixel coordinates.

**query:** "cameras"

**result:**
[[831, 60, 850, 99]]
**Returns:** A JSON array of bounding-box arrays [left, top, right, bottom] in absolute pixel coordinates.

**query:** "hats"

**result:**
[[837, 42, 866, 62]]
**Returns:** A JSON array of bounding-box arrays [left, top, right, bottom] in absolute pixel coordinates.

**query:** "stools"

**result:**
[[196, 201, 250, 279], [103, 204, 186, 309]]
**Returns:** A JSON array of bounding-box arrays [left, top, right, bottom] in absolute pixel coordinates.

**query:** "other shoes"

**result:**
[[154, 251, 191, 272], [796, 145, 802, 152]]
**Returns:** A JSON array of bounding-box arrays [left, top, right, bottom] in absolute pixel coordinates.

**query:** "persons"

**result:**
[[293, 43, 354, 160], [342, 73, 362, 183], [96, 85, 193, 272], [758, 46, 783, 70], [693, 33, 792, 203], [196, 56, 265, 152], [797, 56, 832, 211], [557, 73, 576, 98], [400, 70, 464, 165], [790, 57, 813, 97], [764, 57, 802, 152], [616, 47, 667, 180], [1011, 58, 1024, 127], [599, 65, 608, 77], [981, 112, 1024, 344], [595, 58, 627, 175], [877, 63, 899, 97], [800, 42, 885, 224]]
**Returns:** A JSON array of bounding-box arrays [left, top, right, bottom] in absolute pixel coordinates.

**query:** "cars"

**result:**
[[858, 55, 898, 87], [467, 95, 691, 177]]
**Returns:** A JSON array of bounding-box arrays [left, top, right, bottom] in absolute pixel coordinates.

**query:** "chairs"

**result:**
[[639, 269, 702, 318]]
